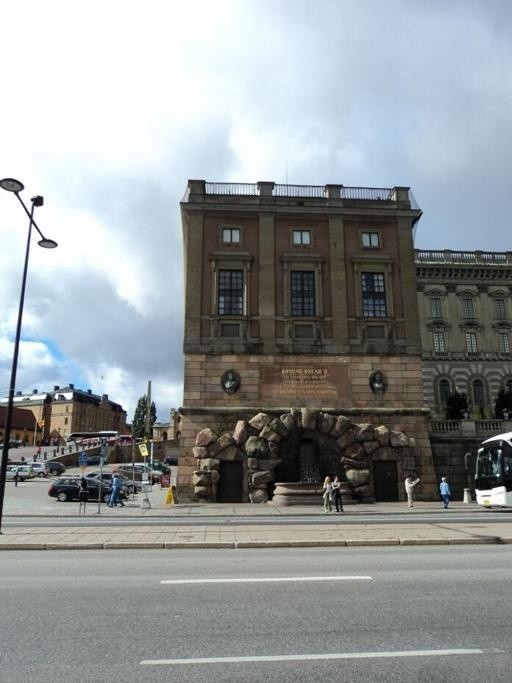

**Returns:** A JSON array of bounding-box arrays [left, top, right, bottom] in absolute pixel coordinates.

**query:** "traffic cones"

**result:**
[[165, 487, 173, 504]]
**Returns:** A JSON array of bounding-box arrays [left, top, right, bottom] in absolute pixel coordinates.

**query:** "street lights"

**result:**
[[0, 178, 58, 530]]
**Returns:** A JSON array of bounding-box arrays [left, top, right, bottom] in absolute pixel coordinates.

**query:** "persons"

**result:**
[[323, 476, 332, 513], [7, 447, 42, 462], [106, 469, 118, 506], [77, 477, 89, 513], [109, 474, 124, 508], [37, 436, 111, 446], [370, 373, 386, 393], [439, 476, 451, 508], [404, 475, 420, 510], [223, 373, 236, 392], [331, 476, 345, 512]]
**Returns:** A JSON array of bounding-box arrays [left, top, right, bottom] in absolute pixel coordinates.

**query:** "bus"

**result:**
[[66, 430, 144, 446], [464, 432, 512, 508]]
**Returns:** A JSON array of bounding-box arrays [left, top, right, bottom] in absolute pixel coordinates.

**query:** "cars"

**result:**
[[164, 455, 178, 465], [48, 458, 171, 502], [0, 440, 25, 449], [6, 462, 65, 482]]
